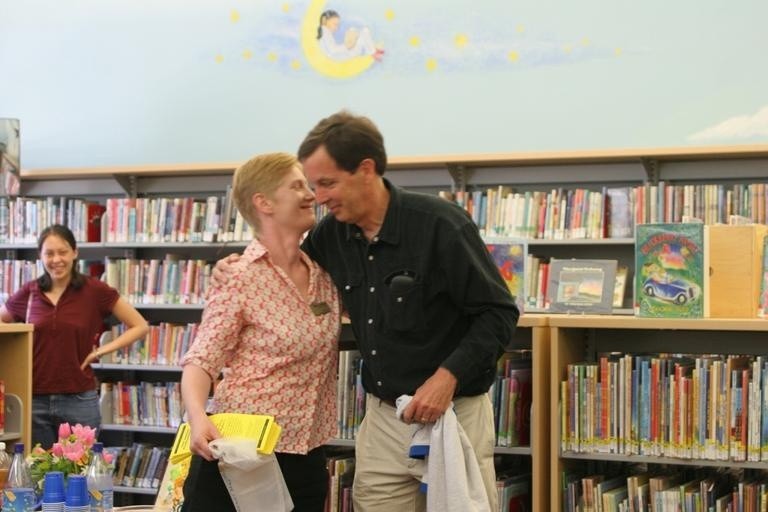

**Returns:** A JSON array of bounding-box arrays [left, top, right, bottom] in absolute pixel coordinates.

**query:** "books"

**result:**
[[110, 320, 199, 367], [93, 376, 187, 429], [481, 223, 768, 318], [489, 347, 767, 462], [495, 472, 767, 509], [436, 184, 766, 240], [105, 442, 173, 488], [0, 194, 331, 243], [325, 348, 368, 509], [1, 252, 213, 305]]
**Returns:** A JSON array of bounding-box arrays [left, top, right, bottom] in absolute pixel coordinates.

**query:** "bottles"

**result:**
[[85, 442, 114, 512], [0, 440, 37, 511]]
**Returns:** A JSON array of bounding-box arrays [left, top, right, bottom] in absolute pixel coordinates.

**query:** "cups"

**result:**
[[41, 470, 91, 512]]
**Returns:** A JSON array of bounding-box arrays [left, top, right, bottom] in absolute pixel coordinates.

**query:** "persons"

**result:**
[[178, 151, 349, 510], [207, 106, 519, 510], [316, 8, 385, 64], [1, 224, 149, 451]]
